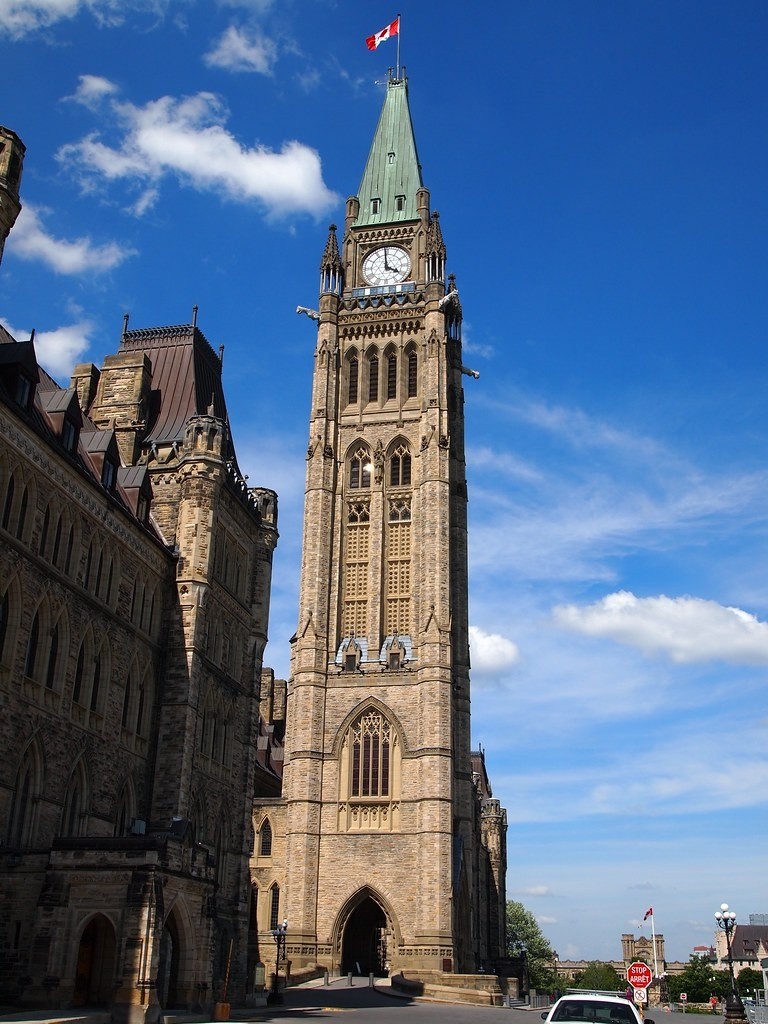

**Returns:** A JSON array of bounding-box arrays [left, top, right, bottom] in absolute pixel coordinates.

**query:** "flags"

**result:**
[[366, 19, 398, 50], [644, 908, 653, 920]]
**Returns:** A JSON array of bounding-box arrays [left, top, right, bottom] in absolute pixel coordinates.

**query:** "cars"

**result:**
[[743, 1000, 756, 1006]]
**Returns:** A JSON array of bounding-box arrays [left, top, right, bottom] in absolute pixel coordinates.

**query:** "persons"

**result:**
[[710, 995, 717, 1014], [720, 995, 727, 1016]]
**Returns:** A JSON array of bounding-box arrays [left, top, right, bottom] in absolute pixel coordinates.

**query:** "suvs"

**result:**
[[541, 988, 655, 1024]]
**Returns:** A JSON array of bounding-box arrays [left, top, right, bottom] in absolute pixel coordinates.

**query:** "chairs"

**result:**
[[610, 1009, 626, 1019], [567, 1005, 583, 1016]]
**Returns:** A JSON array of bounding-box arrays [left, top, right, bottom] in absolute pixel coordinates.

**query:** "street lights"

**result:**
[[266, 924, 286, 1007], [714, 903, 748, 1024]]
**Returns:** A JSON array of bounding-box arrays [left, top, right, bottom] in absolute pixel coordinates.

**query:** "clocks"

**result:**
[[359, 243, 412, 286]]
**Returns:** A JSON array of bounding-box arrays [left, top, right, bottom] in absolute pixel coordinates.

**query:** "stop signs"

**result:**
[[680, 993, 687, 999], [627, 962, 653, 989]]
